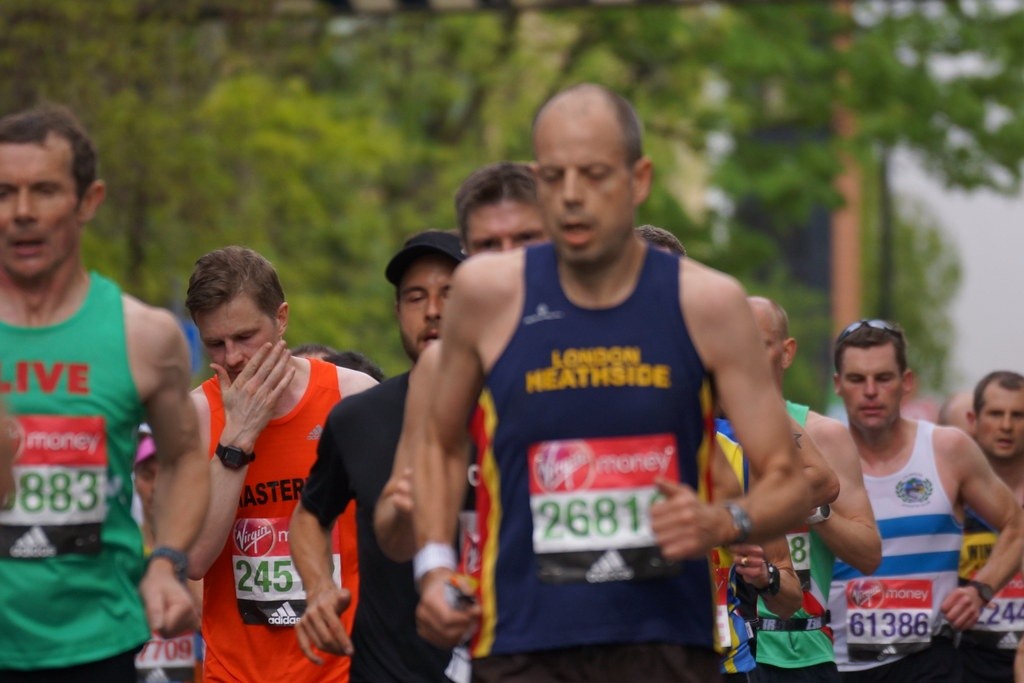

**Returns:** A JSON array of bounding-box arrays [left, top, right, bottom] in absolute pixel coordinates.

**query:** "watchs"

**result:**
[[969, 579, 993, 605], [215, 442, 256, 470], [808, 504, 831, 523], [759, 564, 780, 596]]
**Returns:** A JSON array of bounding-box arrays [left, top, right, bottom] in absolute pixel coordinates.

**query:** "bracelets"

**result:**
[[725, 504, 751, 541], [140, 545, 189, 579], [415, 546, 455, 579]]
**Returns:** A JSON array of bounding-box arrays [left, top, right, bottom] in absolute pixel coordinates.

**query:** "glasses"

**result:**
[[835, 318, 893, 343]]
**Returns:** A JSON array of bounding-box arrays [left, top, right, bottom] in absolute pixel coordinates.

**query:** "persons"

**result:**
[[375, 161, 743, 683], [288, 230, 467, 683], [131, 427, 207, 683], [190, 247, 380, 683], [715, 298, 882, 683], [291, 343, 385, 383], [412, 83, 812, 682], [636, 224, 839, 683], [828, 318, 1024, 683], [938, 371, 1024, 683], [1, 106, 212, 683]]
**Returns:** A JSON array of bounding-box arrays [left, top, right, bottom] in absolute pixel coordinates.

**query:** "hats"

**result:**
[[385, 233, 466, 288]]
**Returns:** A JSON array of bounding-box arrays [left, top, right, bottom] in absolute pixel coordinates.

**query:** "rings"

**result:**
[[741, 557, 749, 566]]
